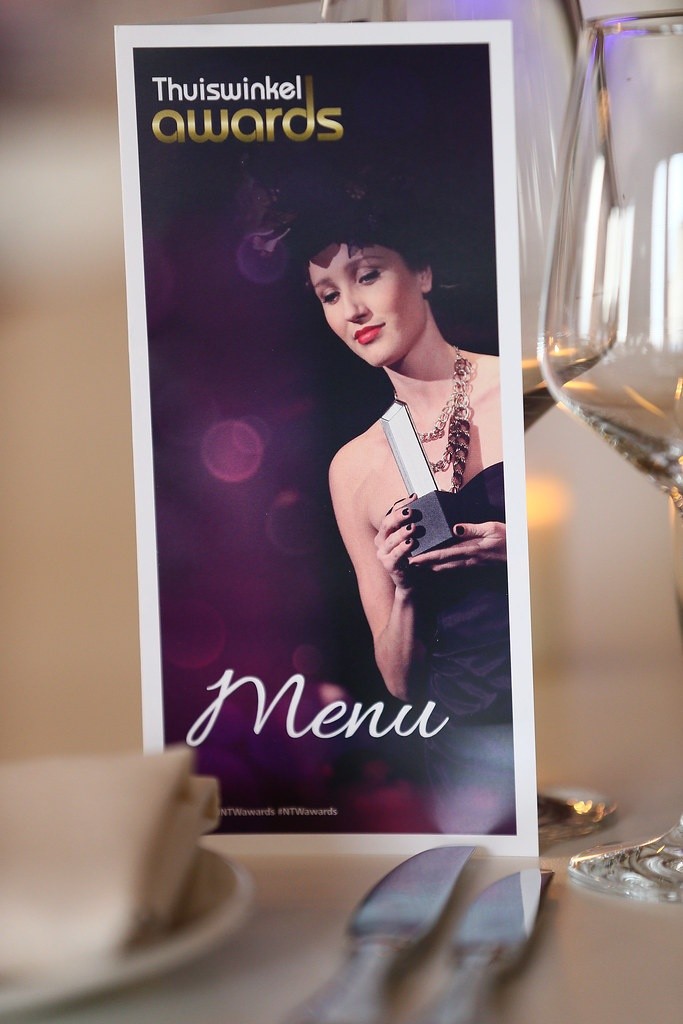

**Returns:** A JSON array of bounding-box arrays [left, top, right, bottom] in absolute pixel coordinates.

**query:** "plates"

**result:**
[[0, 846, 251, 1024]]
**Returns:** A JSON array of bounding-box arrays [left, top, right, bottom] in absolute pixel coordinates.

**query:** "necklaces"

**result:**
[[394, 343, 471, 496]]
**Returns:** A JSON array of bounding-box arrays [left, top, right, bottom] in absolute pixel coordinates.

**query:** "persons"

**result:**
[[286, 190, 516, 835]]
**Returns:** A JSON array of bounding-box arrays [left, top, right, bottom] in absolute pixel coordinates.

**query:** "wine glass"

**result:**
[[537, 16, 683, 910], [309, 0, 623, 860]]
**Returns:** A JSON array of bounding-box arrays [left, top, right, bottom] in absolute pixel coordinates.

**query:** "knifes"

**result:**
[[284, 848, 555, 1024]]
